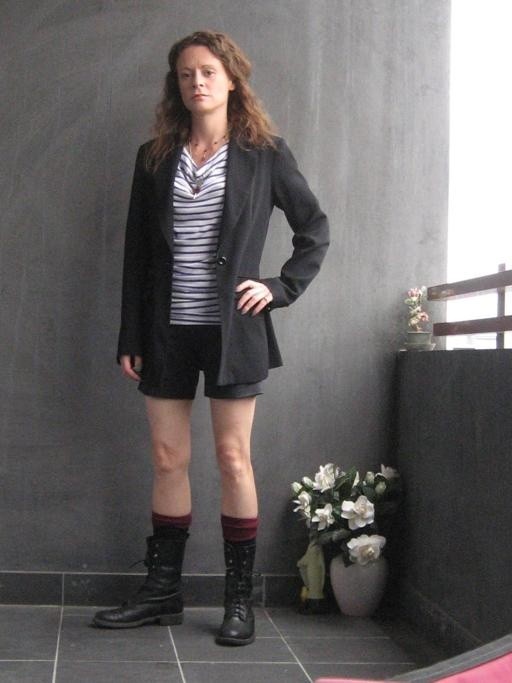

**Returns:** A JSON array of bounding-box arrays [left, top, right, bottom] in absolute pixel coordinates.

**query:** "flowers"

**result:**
[[404, 285, 434, 331], [291, 463, 401, 568]]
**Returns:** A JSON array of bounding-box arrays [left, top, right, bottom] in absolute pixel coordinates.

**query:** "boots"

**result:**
[[93, 524, 192, 631], [215, 537, 256, 644]]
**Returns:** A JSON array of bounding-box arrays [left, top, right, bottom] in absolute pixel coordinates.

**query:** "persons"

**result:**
[[91, 23, 330, 650]]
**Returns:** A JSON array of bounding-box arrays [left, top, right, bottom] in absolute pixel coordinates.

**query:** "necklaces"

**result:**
[[187, 135, 228, 193]]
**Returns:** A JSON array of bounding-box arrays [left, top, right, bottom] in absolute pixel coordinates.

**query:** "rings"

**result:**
[[264, 297, 270, 304]]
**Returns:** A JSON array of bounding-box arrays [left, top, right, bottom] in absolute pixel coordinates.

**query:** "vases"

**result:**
[[406, 330, 432, 346], [328, 548, 391, 618]]
